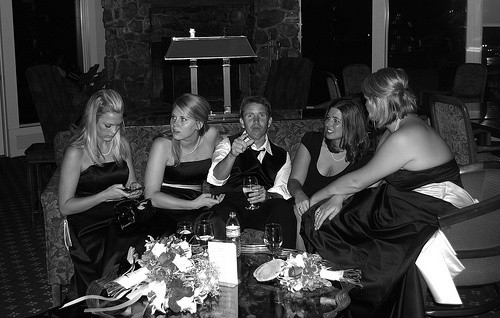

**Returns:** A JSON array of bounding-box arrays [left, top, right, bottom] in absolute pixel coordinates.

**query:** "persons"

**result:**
[[299, 67, 464, 318], [207, 95, 297, 249], [287, 99, 369, 251], [59, 90, 143, 301], [143, 95, 225, 241]]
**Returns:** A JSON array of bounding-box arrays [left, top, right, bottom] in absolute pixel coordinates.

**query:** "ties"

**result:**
[[252, 148, 265, 159]]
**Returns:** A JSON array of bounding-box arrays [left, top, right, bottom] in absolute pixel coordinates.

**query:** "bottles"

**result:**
[[226, 212, 241, 258]]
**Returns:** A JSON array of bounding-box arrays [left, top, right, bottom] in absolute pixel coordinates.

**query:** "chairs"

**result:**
[[407, 59, 500, 318], [324, 64, 370, 99]]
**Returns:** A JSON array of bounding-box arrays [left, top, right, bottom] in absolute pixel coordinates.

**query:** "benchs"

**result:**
[[39, 118, 324, 307]]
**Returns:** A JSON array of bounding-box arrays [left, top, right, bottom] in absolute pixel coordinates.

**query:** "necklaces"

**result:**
[[98, 149, 110, 155], [180, 135, 200, 154], [331, 152, 346, 161]]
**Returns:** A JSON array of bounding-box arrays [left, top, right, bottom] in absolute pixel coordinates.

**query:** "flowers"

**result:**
[[277, 252, 362, 292], [59, 235, 220, 315]]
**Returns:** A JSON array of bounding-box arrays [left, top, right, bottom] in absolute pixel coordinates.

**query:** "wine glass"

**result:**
[[264, 223, 283, 261], [195, 221, 213, 259], [243, 175, 260, 210]]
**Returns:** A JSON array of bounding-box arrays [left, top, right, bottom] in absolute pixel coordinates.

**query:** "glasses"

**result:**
[[244, 112, 269, 120]]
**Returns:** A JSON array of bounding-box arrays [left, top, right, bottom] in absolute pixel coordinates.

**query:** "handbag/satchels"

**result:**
[[114, 199, 156, 235]]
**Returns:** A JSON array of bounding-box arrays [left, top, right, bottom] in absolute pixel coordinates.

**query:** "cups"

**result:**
[[176, 222, 194, 245]]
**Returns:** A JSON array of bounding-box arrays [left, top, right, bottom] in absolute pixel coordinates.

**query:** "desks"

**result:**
[[470, 119, 500, 147], [84, 247, 356, 318], [25, 142, 55, 228]]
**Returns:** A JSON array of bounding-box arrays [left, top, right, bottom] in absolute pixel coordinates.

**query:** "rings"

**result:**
[[299, 208, 302, 210], [317, 212, 320, 215], [258, 195, 261, 199]]
